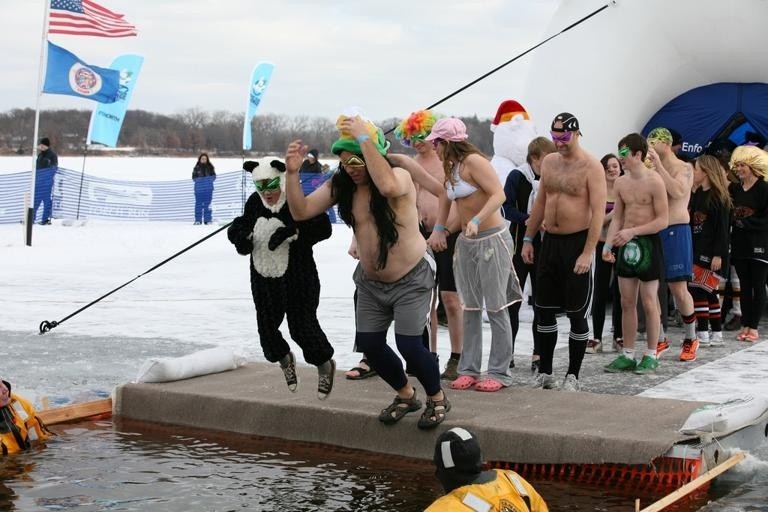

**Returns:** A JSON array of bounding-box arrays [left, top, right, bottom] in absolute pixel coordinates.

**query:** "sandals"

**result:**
[[346, 359, 377, 379], [379, 388, 419, 422], [417, 393, 451, 428]]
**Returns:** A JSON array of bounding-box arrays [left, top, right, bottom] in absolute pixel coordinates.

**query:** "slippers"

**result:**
[[450, 376, 478, 389], [475, 377, 503, 391]]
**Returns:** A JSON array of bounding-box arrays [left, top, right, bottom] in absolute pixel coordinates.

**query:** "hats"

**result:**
[[332, 116, 386, 157], [647, 129, 673, 143], [552, 114, 582, 137], [424, 117, 469, 141], [40, 137, 50, 146], [494, 102, 531, 123], [434, 427, 483, 478]]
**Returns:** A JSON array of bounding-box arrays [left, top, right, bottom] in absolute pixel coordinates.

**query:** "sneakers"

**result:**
[[745, 333, 759, 341], [585, 339, 603, 354], [559, 376, 578, 393], [611, 339, 625, 351], [711, 334, 724, 345], [735, 332, 746, 341], [680, 338, 700, 361], [696, 334, 710, 347], [526, 369, 554, 388], [316, 361, 334, 400], [441, 357, 461, 380], [283, 352, 299, 392], [635, 355, 659, 373], [656, 339, 669, 356], [604, 354, 637, 372]]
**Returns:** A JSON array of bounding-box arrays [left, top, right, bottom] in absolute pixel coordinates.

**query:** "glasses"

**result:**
[[255, 177, 281, 194], [338, 154, 364, 168], [619, 147, 630, 159], [551, 132, 572, 144]]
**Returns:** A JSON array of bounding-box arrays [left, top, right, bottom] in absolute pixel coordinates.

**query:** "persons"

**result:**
[[601, 133, 670, 375], [32, 138, 59, 225], [0, 380, 56, 453], [300, 150, 324, 174], [423, 426, 549, 511], [424, 117, 526, 391], [226, 157, 337, 401], [284, 116, 451, 429], [523, 113, 607, 391], [192, 153, 216, 226], [498, 135, 557, 370], [729, 144, 768, 343], [584, 153, 627, 356], [690, 140, 734, 348], [345, 229, 417, 379], [395, 110, 462, 379], [646, 128, 699, 365]]
